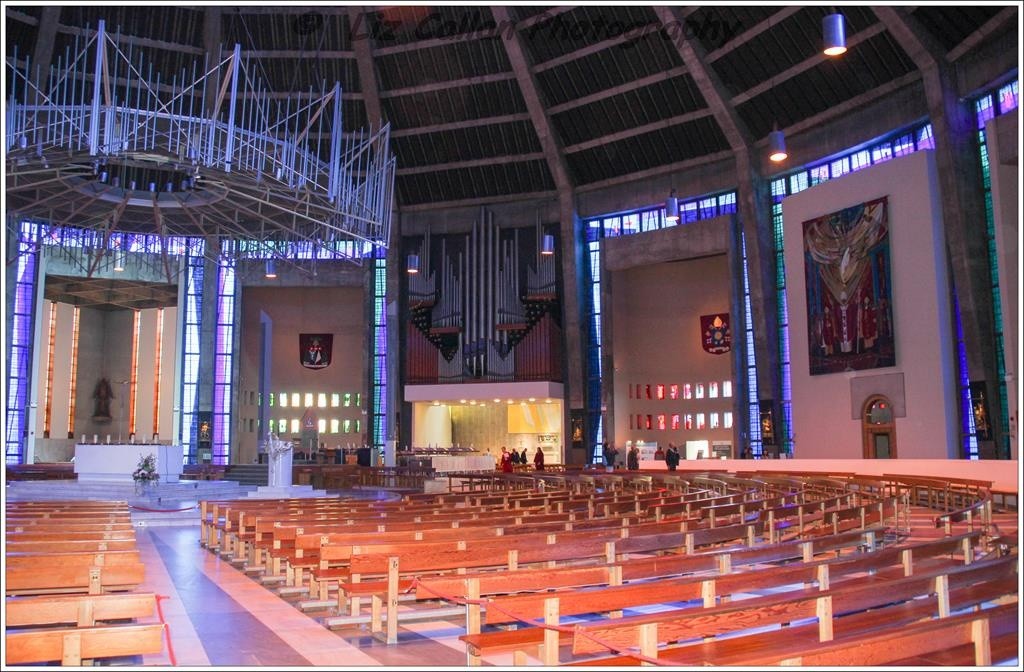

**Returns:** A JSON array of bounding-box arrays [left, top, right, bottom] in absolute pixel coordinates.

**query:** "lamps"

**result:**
[[407, 180, 417, 274], [113, 251, 125, 273], [266, 260, 278, 278], [766, 6, 787, 161], [822, 7, 848, 55], [664, 106, 679, 221], [542, 138, 554, 255]]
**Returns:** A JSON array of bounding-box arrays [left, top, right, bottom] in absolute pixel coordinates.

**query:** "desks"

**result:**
[[408, 455, 498, 478], [75, 442, 183, 482]]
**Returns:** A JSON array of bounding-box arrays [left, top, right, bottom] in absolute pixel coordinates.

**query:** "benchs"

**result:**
[[199, 465, 1019, 667], [5, 501, 163, 667]]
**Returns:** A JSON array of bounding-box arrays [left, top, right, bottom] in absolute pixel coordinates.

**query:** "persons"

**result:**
[[92, 377, 115, 417], [511, 447, 520, 465], [665, 442, 680, 472], [760, 449, 768, 459], [654, 446, 665, 460], [740, 447, 753, 459], [521, 448, 528, 464], [627, 444, 641, 470], [534, 447, 544, 471], [500, 446, 514, 473], [602, 442, 619, 467]]
